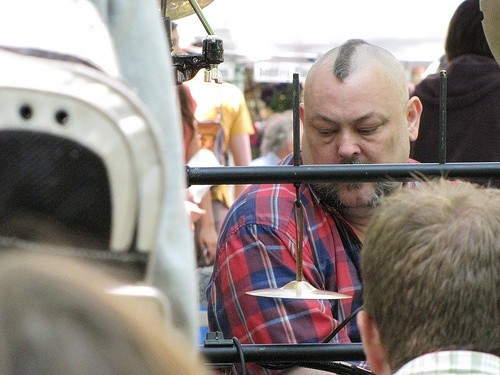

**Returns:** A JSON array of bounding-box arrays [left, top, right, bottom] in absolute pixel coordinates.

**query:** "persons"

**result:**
[[0, 248, 218, 375], [170, 0, 500, 267], [357, 177, 500, 375], [206, 40, 444, 375]]
